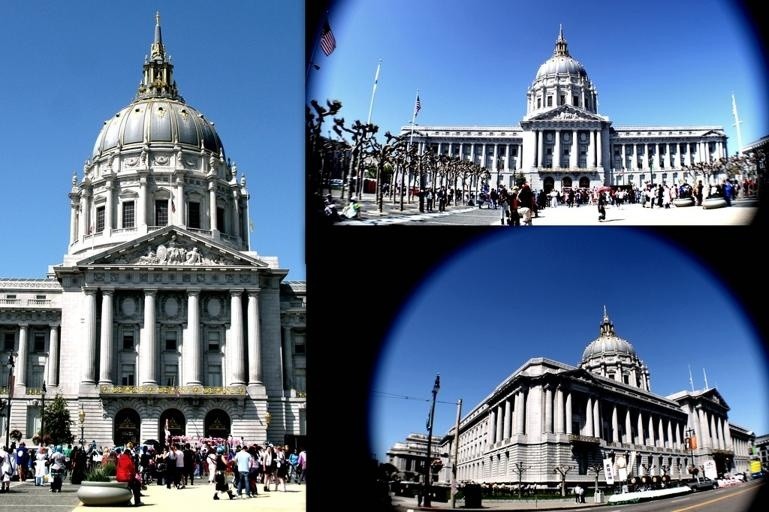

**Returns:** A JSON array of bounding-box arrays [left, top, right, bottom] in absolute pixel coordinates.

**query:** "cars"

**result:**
[[687, 476, 719, 493]]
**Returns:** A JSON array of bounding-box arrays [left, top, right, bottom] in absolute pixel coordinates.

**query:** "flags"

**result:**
[[372, 63, 381, 94], [317, 15, 336, 57], [415, 92, 422, 117]]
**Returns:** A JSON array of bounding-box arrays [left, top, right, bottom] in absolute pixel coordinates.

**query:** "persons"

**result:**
[[137, 234, 201, 265], [425, 177, 759, 226], [574, 483, 582, 502], [0, 432, 307, 507], [416, 482, 425, 506], [554, 108, 579, 120], [579, 483, 586, 504]]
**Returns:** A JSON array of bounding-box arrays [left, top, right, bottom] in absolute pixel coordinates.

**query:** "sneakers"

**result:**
[[263, 486, 289, 493], [50, 488, 62, 493], [211, 490, 260, 500], [0, 486, 11, 494], [135, 493, 146, 507]]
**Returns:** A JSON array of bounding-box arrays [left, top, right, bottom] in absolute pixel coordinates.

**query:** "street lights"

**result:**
[[685, 427, 696, 478]]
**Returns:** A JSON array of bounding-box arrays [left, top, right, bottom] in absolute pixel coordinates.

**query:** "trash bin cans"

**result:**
[[465, 484, 481, 508]]
